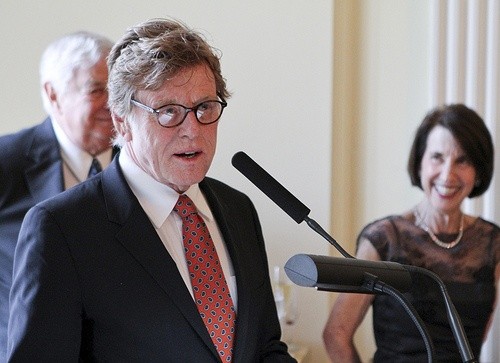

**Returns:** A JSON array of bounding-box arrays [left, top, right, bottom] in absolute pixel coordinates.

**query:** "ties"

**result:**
[[174, 194, 236, 363], [89, 159, 103, 180]]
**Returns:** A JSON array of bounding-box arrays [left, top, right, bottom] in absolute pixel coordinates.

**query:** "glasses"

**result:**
[[130, 91, 227, 126]]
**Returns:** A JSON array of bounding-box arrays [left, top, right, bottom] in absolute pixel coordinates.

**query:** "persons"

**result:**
[[6, 18, 297, 363], [0, 30, 122, 363], [322, 103, 500, 363]]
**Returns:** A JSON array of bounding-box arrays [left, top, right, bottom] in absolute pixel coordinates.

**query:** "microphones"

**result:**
[[231, 151, 352, 258]]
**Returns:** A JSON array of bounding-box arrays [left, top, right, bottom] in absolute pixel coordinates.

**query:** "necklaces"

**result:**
[[413, 206, 465, 249]]
